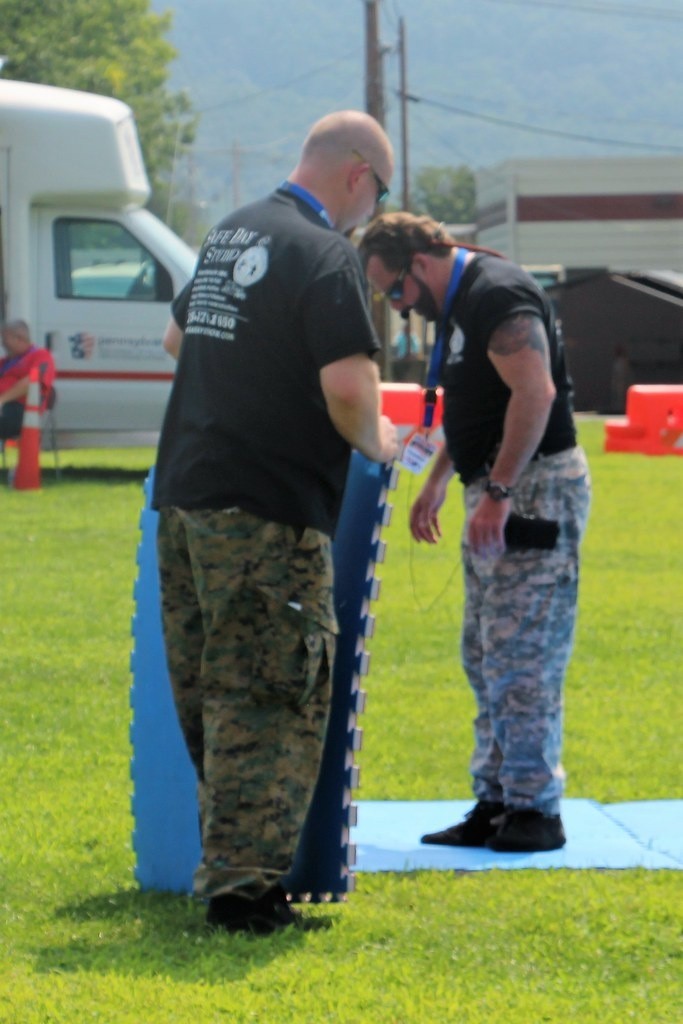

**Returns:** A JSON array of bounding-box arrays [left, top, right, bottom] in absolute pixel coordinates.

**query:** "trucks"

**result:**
[[0, 75, 202, 456]]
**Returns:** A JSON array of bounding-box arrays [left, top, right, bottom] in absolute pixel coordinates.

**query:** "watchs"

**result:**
[[481, 478, 516, 501]]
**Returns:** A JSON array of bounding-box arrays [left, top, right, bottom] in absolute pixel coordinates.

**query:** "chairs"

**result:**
[[0, 386, 62, 487]]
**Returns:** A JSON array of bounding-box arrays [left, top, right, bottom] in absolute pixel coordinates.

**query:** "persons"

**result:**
[[0, 319, 55, 482], [151, 112, 398, 935], [356, 212, 589, 853]]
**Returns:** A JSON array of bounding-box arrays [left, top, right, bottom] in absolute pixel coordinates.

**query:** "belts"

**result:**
[[463, 437, 576, 488]]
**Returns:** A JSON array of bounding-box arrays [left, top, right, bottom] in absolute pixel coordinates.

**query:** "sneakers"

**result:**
[[206, 878, 299, 933], [423, 800, 505, 846], [484, 808, 566, 852]]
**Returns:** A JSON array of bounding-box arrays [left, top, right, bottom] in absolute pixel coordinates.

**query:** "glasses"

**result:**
[[351, 146, 389, 205], [386, 260, 413, 302]]
